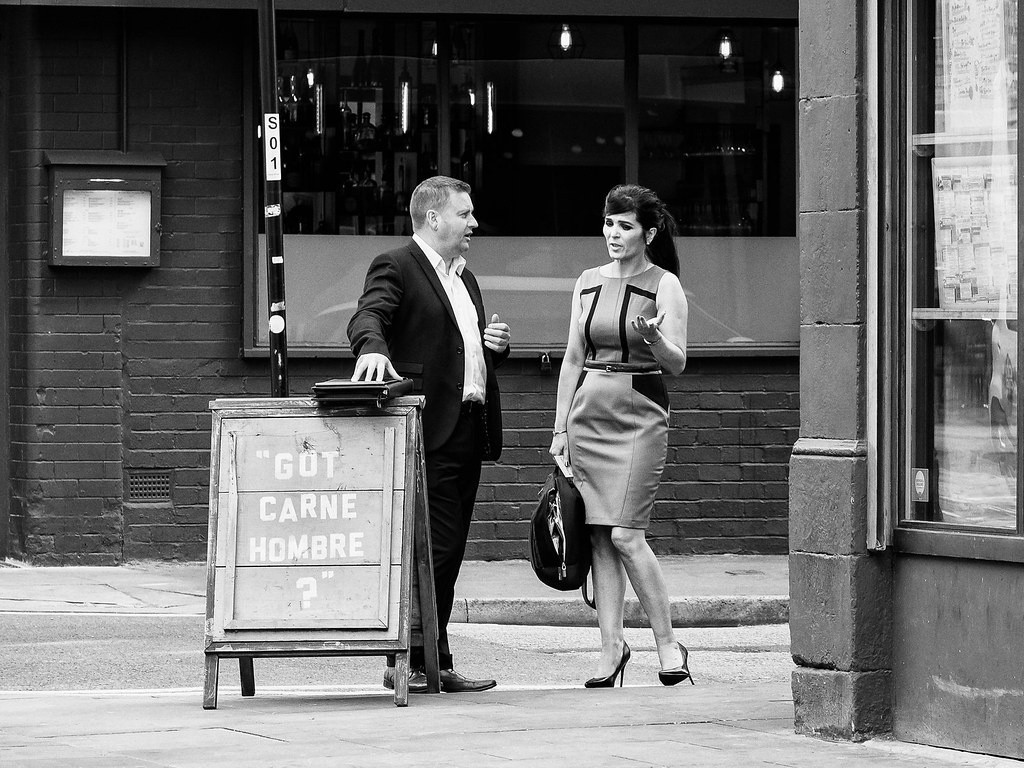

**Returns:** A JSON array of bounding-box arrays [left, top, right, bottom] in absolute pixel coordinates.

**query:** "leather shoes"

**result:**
[[384, 666, 497, 693]]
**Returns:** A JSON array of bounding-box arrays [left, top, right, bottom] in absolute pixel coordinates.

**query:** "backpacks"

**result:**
[[529, 460, 596, 609]]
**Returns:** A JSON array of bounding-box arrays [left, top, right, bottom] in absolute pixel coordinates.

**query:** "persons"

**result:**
[[548, 185, 694, 690], [347, 175, 510, 696]]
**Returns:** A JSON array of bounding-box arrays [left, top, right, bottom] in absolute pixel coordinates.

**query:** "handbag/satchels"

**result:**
[[312, 379, 413, 402]]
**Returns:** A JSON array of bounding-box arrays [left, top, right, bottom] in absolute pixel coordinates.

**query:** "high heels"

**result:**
[[584, 641, 631, 687], [659, 641, 695, 685]]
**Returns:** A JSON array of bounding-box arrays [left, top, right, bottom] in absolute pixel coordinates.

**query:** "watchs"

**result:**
[[552, 430, 567, 437]]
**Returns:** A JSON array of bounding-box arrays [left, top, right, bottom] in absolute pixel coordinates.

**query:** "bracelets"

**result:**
[[642, 336, 661, 346]]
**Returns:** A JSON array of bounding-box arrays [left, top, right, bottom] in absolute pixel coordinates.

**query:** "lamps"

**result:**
[[399, 58, 412, 135], [488, 62, 495, 135], [719, 25, 731, 62], [305, 61, 314, 89], [313, 63, 324, 135], [772, 53, 784, 93], [469, 77, 477, 108], [559, 16, 573, 52]]
